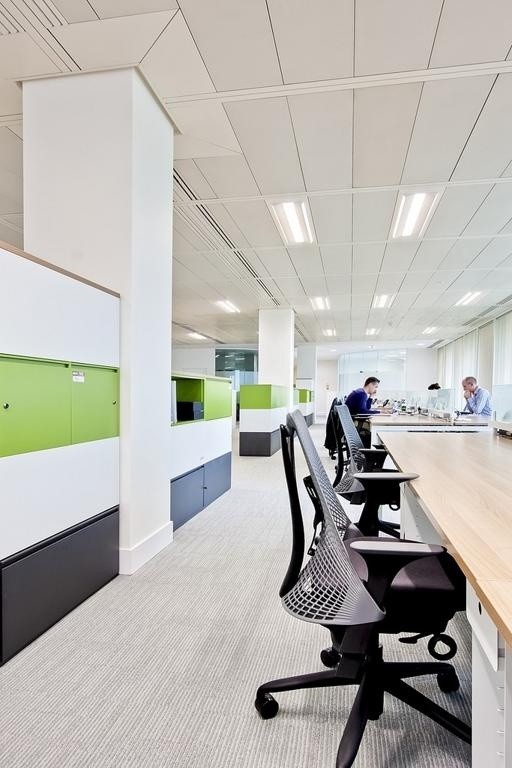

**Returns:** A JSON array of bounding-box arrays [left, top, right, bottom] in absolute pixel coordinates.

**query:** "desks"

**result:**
[[377, 430, 512, 767], [370, 405, 492, 446]]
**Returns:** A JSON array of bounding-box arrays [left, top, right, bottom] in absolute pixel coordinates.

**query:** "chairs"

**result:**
[[331, 406, 421, 538], [255, 409, 471, 768], [330, 400, 371, 488]]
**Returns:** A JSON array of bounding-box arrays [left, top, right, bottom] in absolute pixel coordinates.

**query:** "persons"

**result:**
[[462, 377, 492, 417], [338, 376, 389, 449]]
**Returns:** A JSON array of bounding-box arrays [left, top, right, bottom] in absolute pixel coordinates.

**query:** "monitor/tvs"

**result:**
[[393, 388, 455, 414]]
